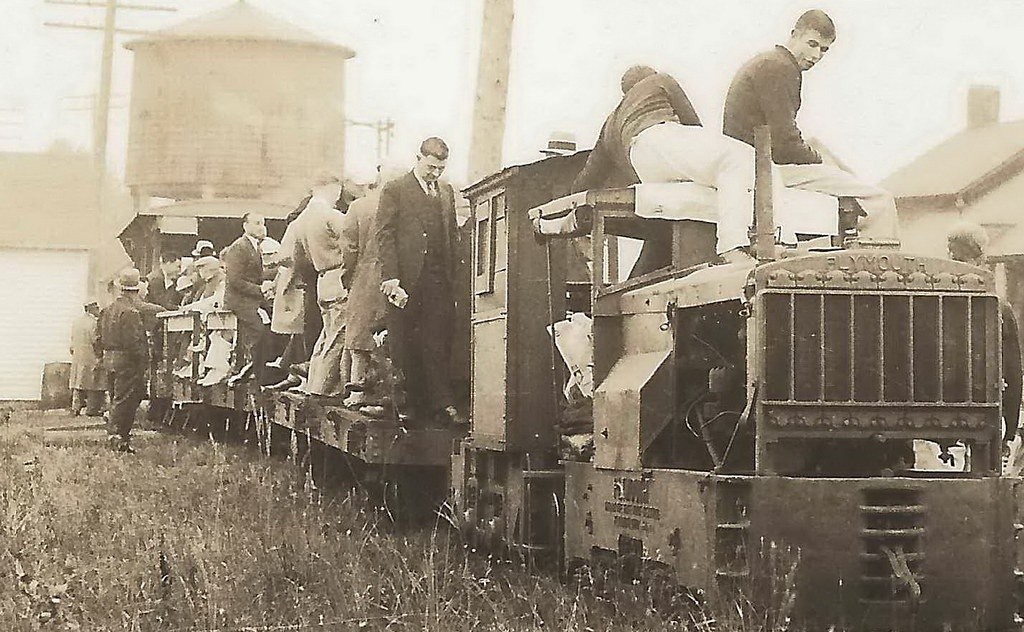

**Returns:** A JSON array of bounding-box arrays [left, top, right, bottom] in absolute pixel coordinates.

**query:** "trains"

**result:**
[[115, 125, 1023, 630]]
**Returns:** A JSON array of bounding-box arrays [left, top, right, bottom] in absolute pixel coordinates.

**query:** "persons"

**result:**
[[70, 161, 391, 456], [569, 67, 800, 255], [373, 137, 464, 426], [723, 9, 898, 242]]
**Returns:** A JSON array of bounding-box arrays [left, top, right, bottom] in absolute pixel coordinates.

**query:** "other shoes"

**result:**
[[203, 367, 233, 387], [708, 247, 758, 267], [437, 405, 468, 429], [344, 377, 366, 391], [73, 410, 81, 416], [360, 406, 383, 416], [197, 369, 214, 385], [113, 440, 136, 455], [86, 410, 104, 416], [266, 357, 283, 368], [290, 362, 310, 377], [289, 382, 307, 392], [266, 378, 298, 391]]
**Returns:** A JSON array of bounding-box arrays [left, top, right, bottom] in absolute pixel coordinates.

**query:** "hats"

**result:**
[[176, 276, 193, 291], [190, 240, 216, 256], [113, 268, 146, 290], [196, 256, 219, 281], [84, 301, 100, 311]]
